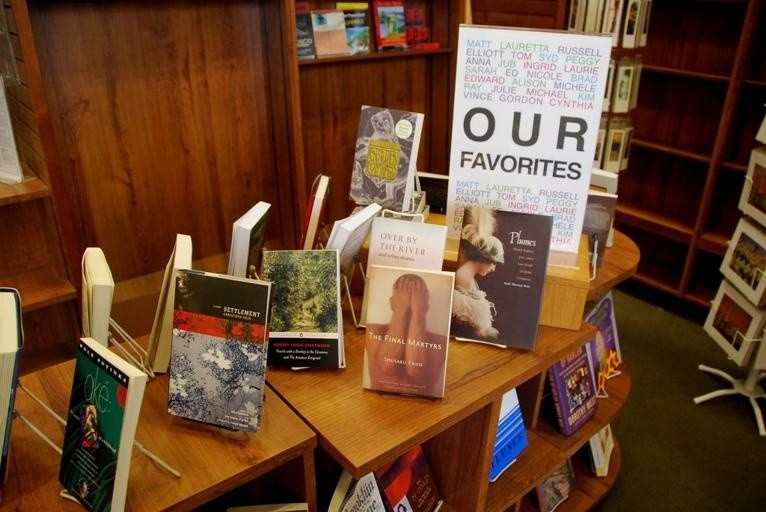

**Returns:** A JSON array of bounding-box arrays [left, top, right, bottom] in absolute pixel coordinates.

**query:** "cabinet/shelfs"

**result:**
[[610, 1, 766, 306], [266, 227, 643, 512], [288, 1, 469, 64], [692, 117, 766, 437], [1, 1, 85, 379]]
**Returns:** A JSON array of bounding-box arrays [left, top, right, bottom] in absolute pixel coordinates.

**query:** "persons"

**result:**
[[592, 326, 605, 361], [452, 206, 509, 341], [365, 271, 446, 396]]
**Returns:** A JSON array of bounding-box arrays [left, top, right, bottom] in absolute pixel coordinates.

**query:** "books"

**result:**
[[347, 104, 425, 211], [0, 287, 25, 490], [702, 113, 765, 370], [261, 249, 347, 373], [80, 246, 115, 349], [324, 202, 381, 272], [325, 467, 389, 512], [56, 334, 147, 512], [224, 199, 274, 278], [566, 1, 656, 174], [536, 455, 579, 512], [299, 171, 333, 250], [148, 232, 193, 374], [164, 266, 273, 435], [489, 387, 528, 480], [374, 443, 445, 511], [357, 211, 450, 332], [582, 289, 622, 393], [581, 188, 621, 269], [546, 346, 598, 435], [586, 164, 620, 249], [587, 424, 614, 478], [295, 0, 441, 60], [358, 262, 456, 401], [445, 204, 553, 352]]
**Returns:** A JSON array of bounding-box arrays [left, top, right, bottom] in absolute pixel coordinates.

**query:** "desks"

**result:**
[[1, 333, 318, 511]]
